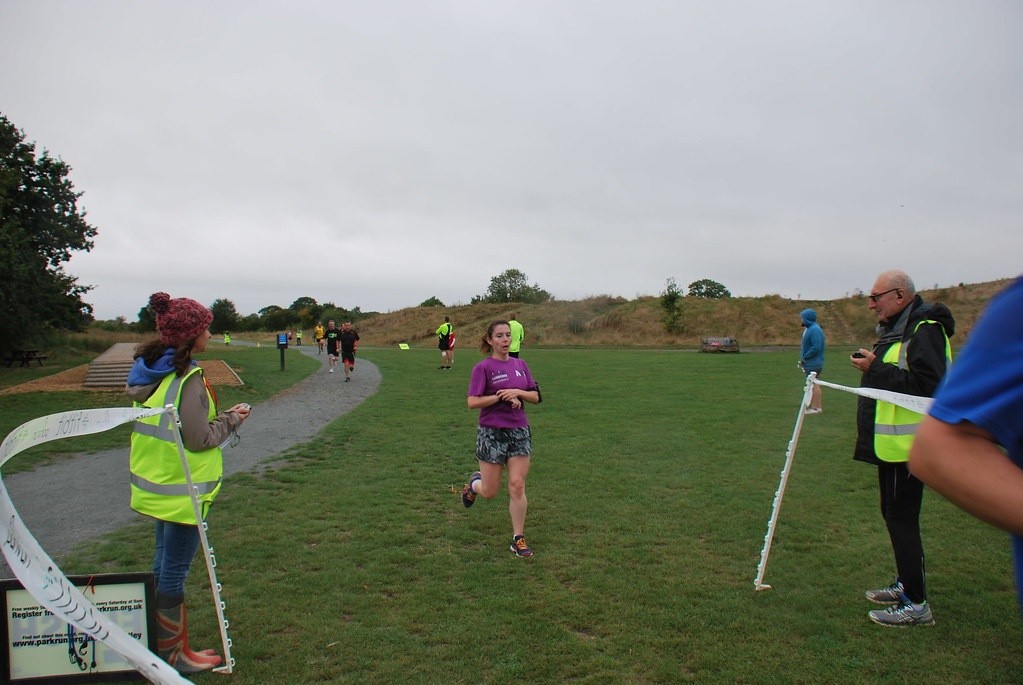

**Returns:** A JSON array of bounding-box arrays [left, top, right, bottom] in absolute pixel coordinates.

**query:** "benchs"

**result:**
[[701, 337, 740, 353]]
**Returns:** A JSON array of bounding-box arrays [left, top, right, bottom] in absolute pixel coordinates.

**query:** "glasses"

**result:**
[[868, 287, 899, 302]]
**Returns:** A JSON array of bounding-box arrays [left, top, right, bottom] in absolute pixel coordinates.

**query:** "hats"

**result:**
[[148, 292, 215, 347]]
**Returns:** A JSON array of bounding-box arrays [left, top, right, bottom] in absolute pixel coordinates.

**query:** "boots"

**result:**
[[154, 603, 222, 674]]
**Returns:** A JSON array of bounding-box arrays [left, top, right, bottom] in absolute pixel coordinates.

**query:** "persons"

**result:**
[[286, 329, 292, 346], [906, 275, 1023, 606], [336, 320, 360, 383], [448, 332, 454, 364], [124, 291, 250, 674], [322, 321, 340, 372], [848, 270, 956, 630], [462, 321, 541, 558], [339, 323, 344, 330], [798, 309, 825, 414], [435, 316, 452, 371], [507, 312, 524, 359], [314, 321, 325, 354], [224, 331, 230, 346], [312, 333, 315, 345], [295, 329, 301, 346]]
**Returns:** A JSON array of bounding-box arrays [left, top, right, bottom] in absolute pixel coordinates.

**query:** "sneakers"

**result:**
[[868, 594, 937, 628], [866, 576, 909, 605]]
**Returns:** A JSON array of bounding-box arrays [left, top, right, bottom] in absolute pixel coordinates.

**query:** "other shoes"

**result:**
[[803, 407, 822, 414], [458, 471, 482, 508], [437, 366, 453, 370], [510, 534, 534, 558], [350, 366, 354, 371], [329, 369, 333, 373], [344, 376, 351, 382]]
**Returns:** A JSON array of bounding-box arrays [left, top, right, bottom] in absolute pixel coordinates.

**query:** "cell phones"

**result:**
[[852, 352, 866, 358], [237, 403, 251, 411]]
